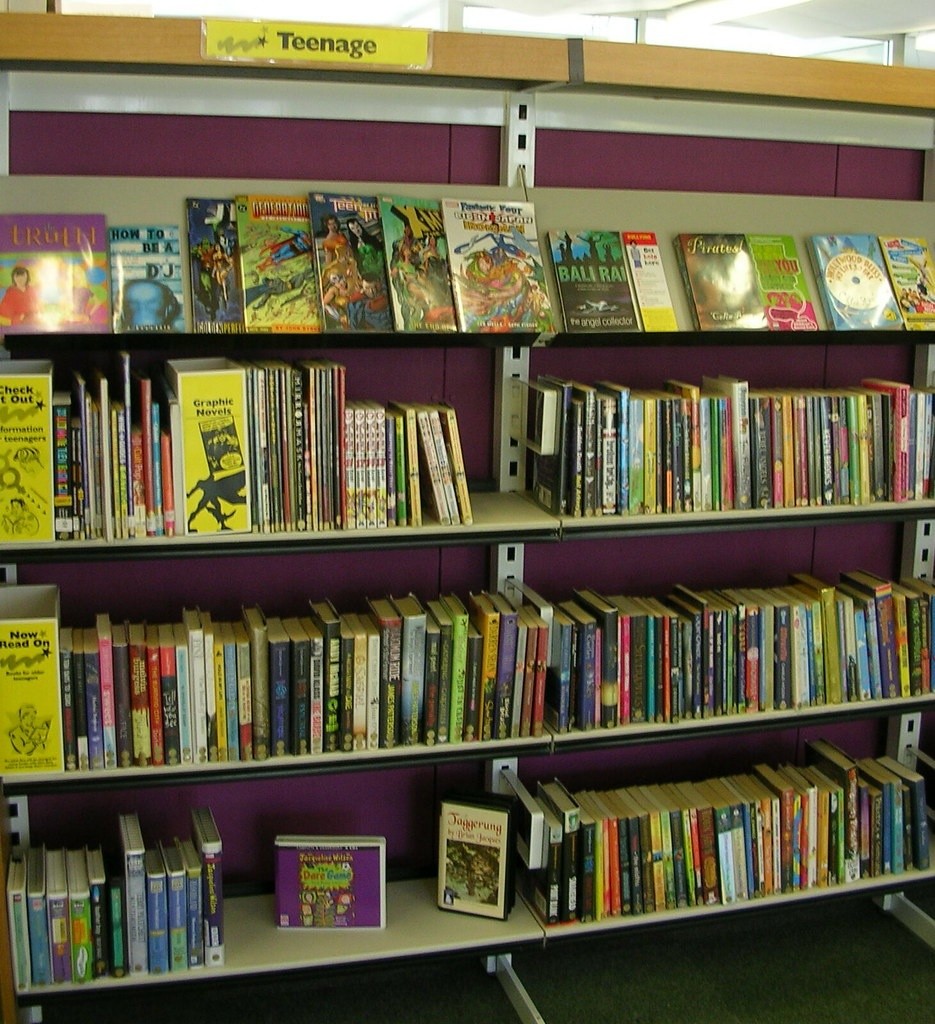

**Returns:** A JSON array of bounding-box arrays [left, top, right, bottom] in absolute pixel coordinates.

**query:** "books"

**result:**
[[0, 193, 935, 993]]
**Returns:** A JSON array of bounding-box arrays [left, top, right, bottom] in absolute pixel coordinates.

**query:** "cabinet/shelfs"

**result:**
[[0, 13, 935, 1024]]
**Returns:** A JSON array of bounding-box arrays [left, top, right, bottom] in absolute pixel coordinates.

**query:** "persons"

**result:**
[[0, 266, 41, 329]]
